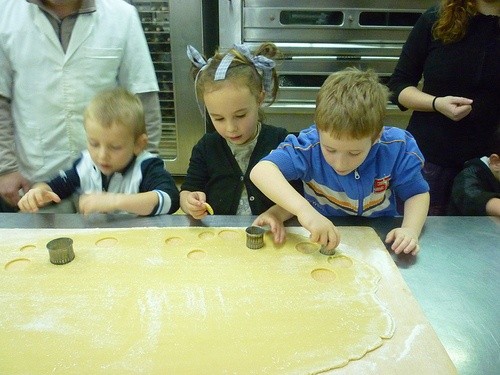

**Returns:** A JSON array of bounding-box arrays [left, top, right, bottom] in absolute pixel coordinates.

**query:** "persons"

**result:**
[[453, 134, 500, 218], [388, 0, 500, 215], [249, 68, 431, 255], [179, 42, 294, 245], [0, 0, 162, 213], [18, 88, 180, 217]]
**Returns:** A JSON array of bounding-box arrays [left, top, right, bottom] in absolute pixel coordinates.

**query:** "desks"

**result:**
[[0, 212, 500, 375]]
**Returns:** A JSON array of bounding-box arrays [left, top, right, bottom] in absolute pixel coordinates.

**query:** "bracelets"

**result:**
[[433, 96, 439, 112]]
